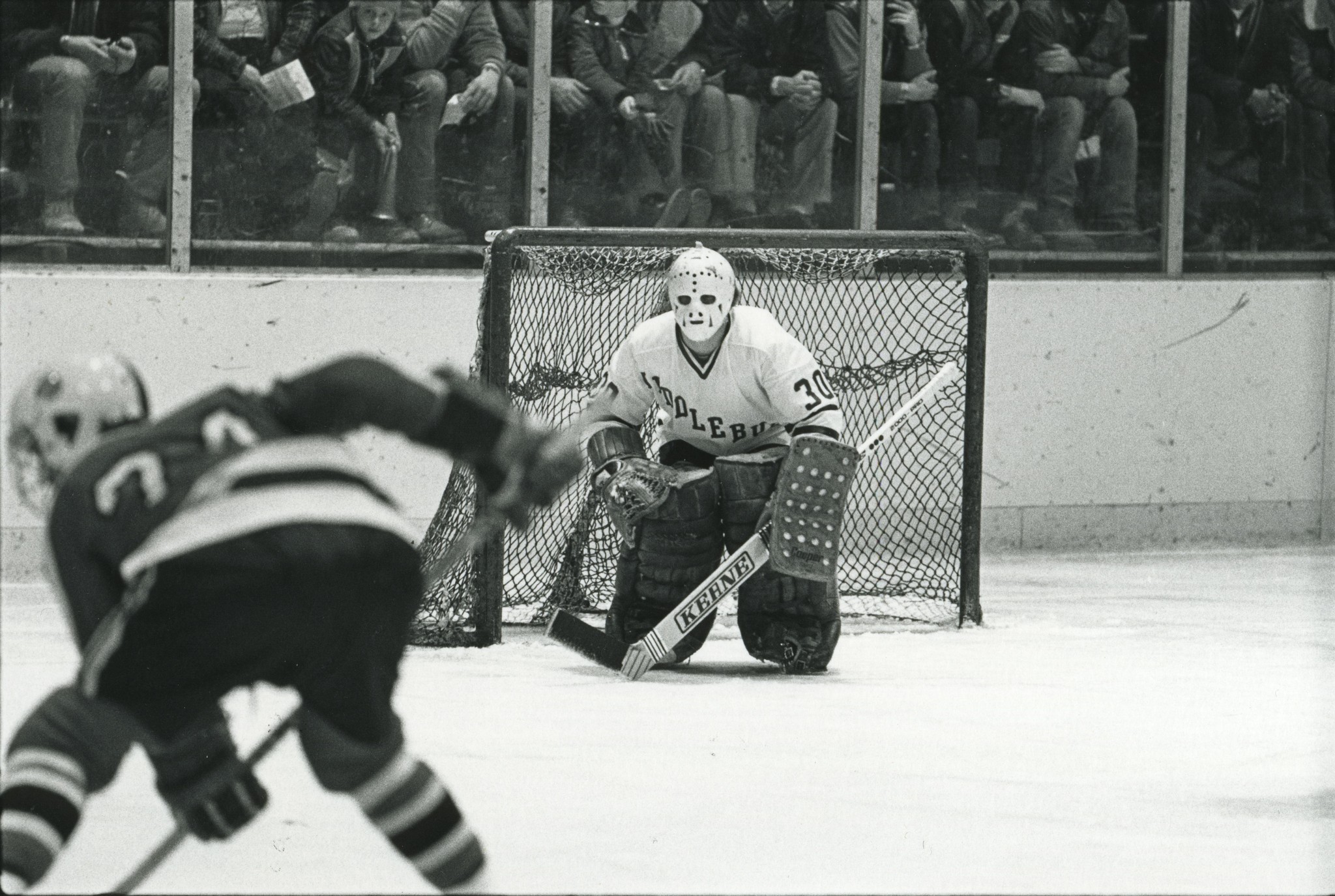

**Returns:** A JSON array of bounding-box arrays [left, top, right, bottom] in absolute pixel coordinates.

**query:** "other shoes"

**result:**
[[357, 213, 421, 243], [445, 218, 516, 245], [104, 196, 169, 237], [1094, 215, 1156, 251], [403, 210, 468, 244], [634, 187, 691, 229], [1036, 206, 1097, 251], [678, 188, 713, 229], [319, 216, 362, 241], [770, 215, 815, 230], [36, 194, 84, 235], [932, 221, 1007, 251], [294, 171, 337, 242], [984, 206, 1047, 252]]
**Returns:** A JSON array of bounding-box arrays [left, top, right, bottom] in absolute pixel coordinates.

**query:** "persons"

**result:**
[[583, 246, 843, 675], [0, 349, 586, 896], [0, 0, 1335, 252]]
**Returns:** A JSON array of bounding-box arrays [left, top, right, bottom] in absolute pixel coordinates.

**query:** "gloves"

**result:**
[[458, 408, 587, 531], [152, 727, 270, 842]]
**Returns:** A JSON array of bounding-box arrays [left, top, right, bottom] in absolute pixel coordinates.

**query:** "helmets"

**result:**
[[7, 351, 148, 519]]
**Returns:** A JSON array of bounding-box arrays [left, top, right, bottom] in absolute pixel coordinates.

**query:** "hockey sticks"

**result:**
[[544, 358, 964, 680], [107, 509, 506, 896]]
